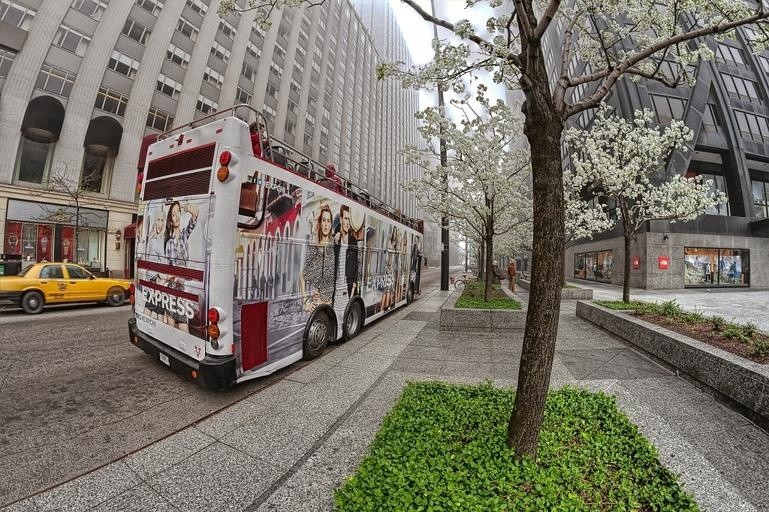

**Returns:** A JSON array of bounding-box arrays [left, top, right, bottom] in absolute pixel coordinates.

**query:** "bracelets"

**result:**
[[303, 299, 308, 305]]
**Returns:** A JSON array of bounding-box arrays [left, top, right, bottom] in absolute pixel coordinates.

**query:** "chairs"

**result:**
[[251, 130, 345, 194]]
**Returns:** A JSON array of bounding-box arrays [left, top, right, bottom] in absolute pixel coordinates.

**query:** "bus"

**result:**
[[128, 103, 424, 393]]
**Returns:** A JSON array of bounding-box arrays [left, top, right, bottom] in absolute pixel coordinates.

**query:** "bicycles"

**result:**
[[454, 274, 470, 289]]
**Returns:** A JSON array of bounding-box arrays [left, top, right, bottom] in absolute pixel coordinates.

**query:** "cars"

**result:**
[[0, 262, 132, 315]]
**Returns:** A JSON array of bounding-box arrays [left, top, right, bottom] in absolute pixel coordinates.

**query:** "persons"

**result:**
[[593, 261, 600, 275], [493, 260, 502, 283], [164, 200, 199, 330], [326, 164, 343, 193], [705, 262, 710, 282], [300, 204, 340, 313], [508, 259, 517, 295], [721, 257, 725, 272], [364, 226, 422, 311], [332, 204, 361, 338], [728, 262, 736, 281], [250, 121, 269, 148], [361, 188, 369, 198], [142, 207, 164, 324]]
